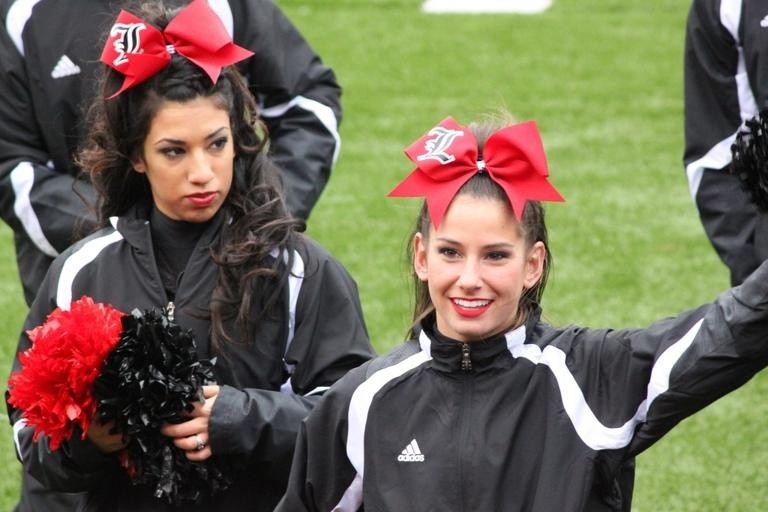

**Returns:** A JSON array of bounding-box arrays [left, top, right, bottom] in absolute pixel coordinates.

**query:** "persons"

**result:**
[[0, 0, 376, 512], [251, 103, 766, 512], [678, 1, 766, 294], [0, 0, 339, 310]]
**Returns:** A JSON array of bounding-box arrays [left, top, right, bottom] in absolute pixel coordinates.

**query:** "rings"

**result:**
[[194, 435, 208, 452]]
